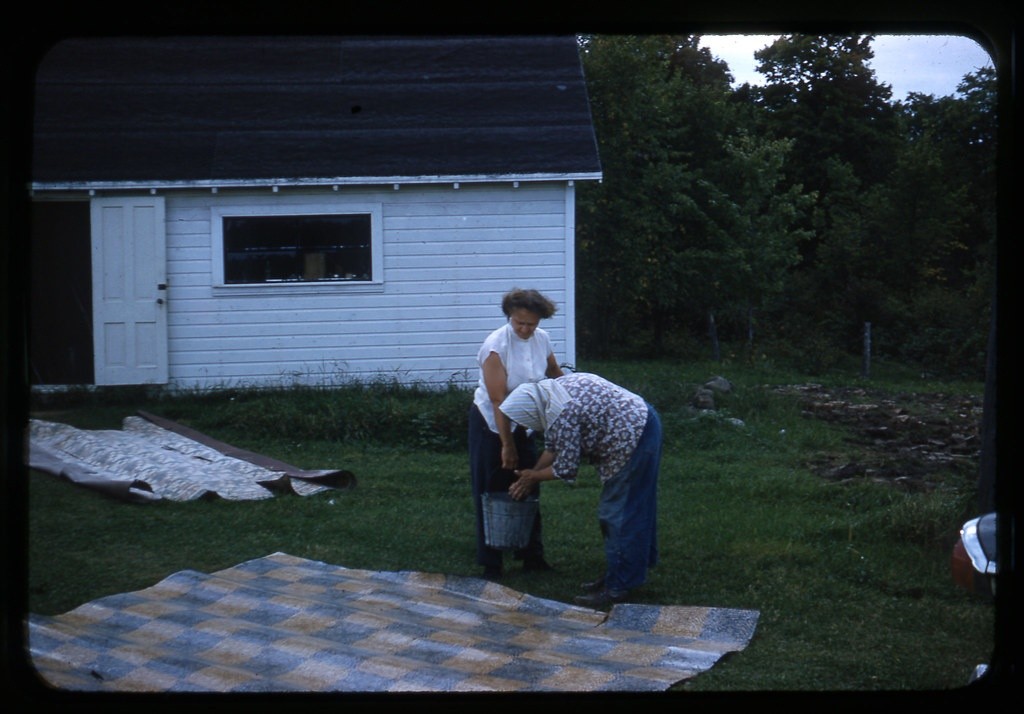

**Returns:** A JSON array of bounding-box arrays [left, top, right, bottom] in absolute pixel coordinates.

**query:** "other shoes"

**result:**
[[574, 588, 630, 604], [481, 566, 507, 582], [580, 574, 606, 593], [514, 551, 551, 572]]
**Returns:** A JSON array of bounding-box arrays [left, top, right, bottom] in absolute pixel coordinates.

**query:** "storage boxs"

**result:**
[[304, 253, 325, 277]]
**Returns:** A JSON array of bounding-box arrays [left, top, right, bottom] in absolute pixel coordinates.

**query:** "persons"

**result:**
[[497, 372, 664, 607], [469, 289, 564, 583]]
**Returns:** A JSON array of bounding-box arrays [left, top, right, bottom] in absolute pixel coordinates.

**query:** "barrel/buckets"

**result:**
[[479, 463, 538, 550]]
